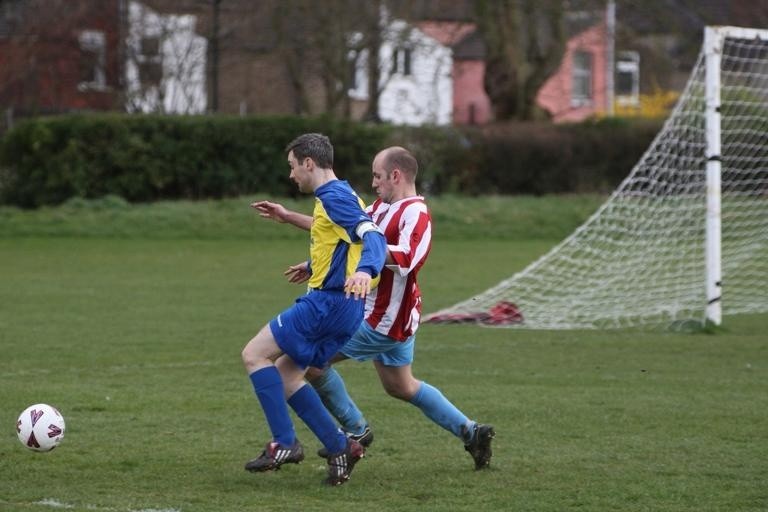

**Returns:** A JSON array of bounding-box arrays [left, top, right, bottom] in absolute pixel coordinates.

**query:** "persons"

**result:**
[[252, 145, 495, 470], [241, 132, 385, 486]]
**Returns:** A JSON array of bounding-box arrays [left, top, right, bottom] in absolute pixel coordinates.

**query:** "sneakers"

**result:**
[[246, 439, 303, 472], [317, 426, 374, 458], [463, 424, 495, 469], [322, 438, 366, 484]]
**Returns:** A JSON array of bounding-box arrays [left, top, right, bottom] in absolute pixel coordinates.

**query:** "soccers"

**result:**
[[16, 403, 65, 452]]
